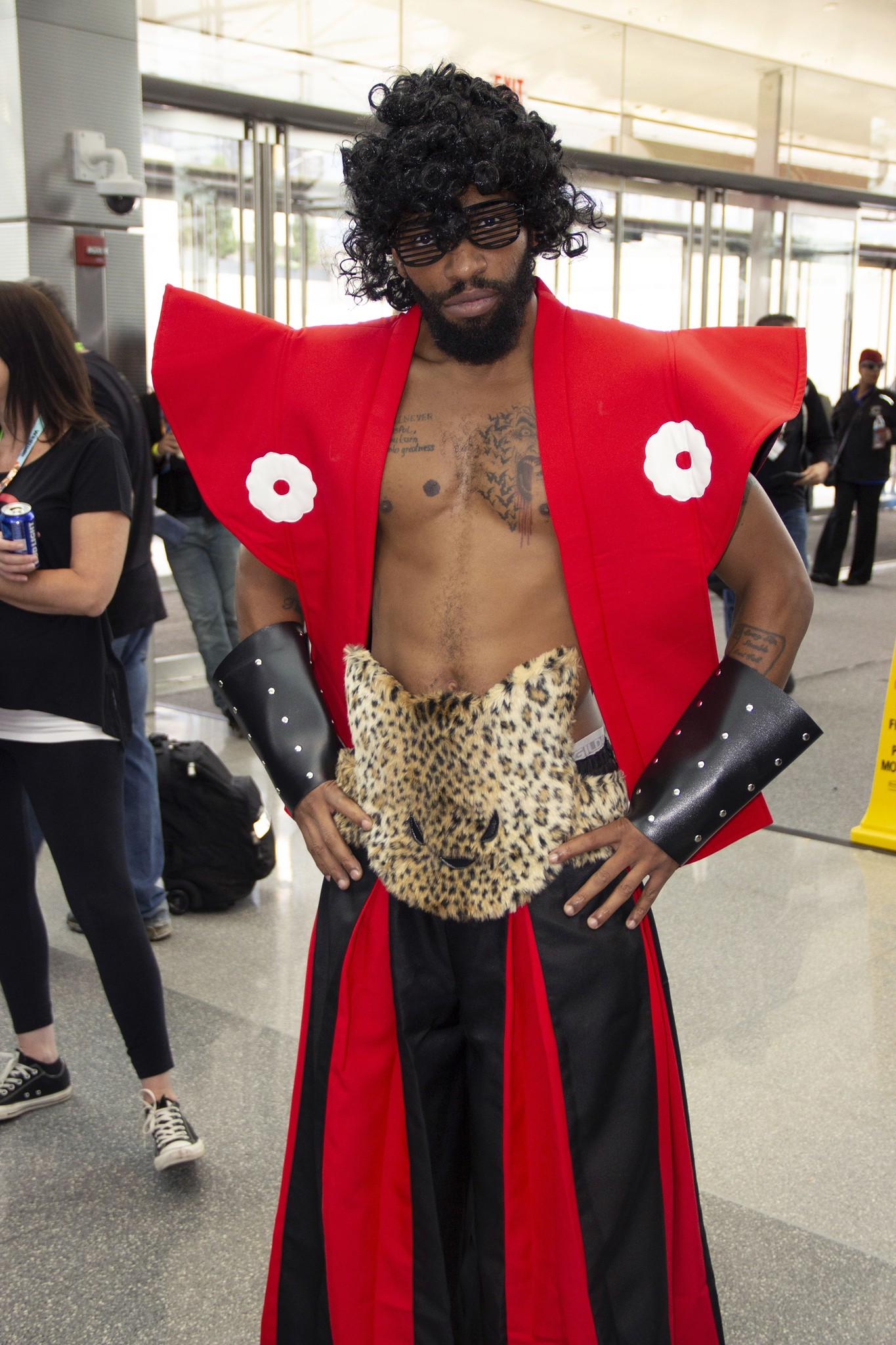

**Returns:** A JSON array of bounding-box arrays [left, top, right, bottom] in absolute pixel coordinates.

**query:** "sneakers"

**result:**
[[143, 1092, 206, 1172], [0, 1050, 72, 1124]]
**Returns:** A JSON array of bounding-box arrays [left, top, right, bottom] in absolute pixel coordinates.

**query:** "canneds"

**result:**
[[0, 502, 39, 571]]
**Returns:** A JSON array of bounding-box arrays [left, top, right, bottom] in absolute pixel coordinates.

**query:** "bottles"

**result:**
[[873, 414, 885, 449]]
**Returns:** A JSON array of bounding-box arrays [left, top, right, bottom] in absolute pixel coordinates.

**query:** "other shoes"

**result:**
[[843, 576, 868, 586], [809, 574, 838, 587], [65, 901, 176, 943]]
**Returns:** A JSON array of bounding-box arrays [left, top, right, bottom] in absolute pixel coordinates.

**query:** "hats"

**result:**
[[861, 349, 882, 362]]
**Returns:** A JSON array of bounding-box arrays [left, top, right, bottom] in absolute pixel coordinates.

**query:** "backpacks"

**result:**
[[150, 733, 277, 913]]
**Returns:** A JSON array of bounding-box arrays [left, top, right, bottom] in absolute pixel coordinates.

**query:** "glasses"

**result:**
[[862, 362, 884, 369], [395, 198, 521, 269]]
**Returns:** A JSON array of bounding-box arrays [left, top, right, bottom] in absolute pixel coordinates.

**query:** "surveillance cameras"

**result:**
[[97, 178, 147, 215]]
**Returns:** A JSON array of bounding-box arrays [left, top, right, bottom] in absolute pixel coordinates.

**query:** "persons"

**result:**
[[21, 276, 240, 941], [723, 314, 896, 693], [0, 281, 205, 1172], [152, 63, 823, 1345]]
